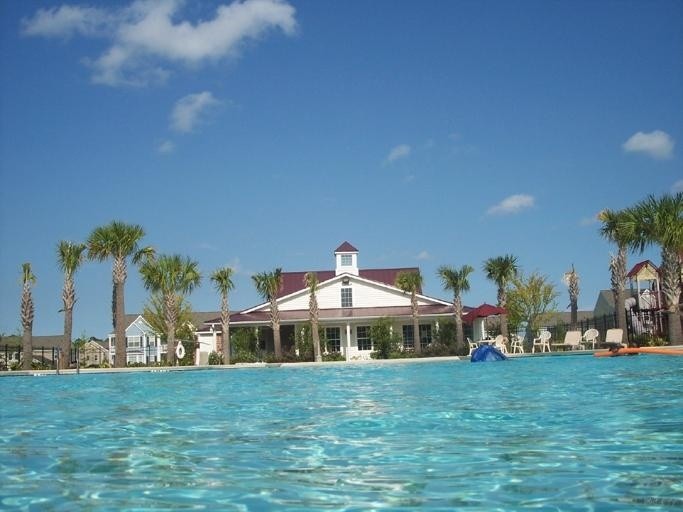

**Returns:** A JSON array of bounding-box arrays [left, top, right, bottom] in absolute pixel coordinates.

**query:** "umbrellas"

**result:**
[[459, 301, 510, 339]]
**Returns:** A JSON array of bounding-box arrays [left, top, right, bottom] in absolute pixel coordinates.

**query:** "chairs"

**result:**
[[467, 328, 627, 355]]
